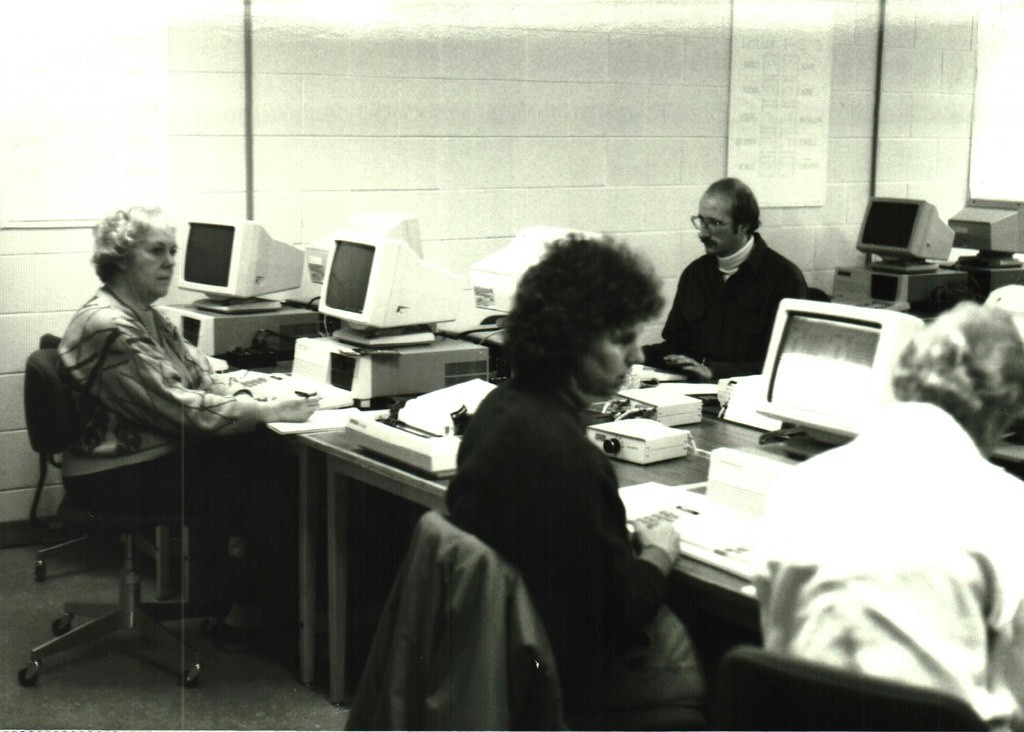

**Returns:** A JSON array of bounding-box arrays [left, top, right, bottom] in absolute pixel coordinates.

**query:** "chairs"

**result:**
[[414, 510, 704, 732], [18, 335, 204, 685], [710, 647, 988, 732]]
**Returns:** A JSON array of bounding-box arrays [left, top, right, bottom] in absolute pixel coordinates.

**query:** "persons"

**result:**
[[56, 208, 322, 649], [447, 233, 743, 732], [751, 301, 1024, 732], [626, 178, 808, 381]]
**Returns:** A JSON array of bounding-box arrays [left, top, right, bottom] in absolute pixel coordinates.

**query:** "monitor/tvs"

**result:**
[[175, 217, 604, 349], [753, 198, 1024, 458]]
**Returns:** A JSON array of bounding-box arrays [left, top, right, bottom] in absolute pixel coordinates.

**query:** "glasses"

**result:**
[[691, 214, 733, 233]]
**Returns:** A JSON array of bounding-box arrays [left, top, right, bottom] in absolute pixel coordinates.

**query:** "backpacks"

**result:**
[[24, 328, 120, 453]]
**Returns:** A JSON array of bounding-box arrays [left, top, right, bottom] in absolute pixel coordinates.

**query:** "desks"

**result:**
[[179, 346, 835, 705]]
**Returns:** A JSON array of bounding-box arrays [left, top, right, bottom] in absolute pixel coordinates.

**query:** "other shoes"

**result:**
[[217, 622, 279, 652]]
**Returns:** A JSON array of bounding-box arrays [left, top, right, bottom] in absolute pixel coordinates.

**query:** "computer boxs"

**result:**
[[158, 303, 320, 359], [292, 334, 491, 400]]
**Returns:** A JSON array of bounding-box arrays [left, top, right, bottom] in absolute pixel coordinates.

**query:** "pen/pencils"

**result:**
[[295, 391, 310, 398]]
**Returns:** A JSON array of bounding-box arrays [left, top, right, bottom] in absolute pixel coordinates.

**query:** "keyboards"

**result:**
[[206, 356, 228, 371], [615, 480, 772, 580], [270, 374, 354, 410]]
